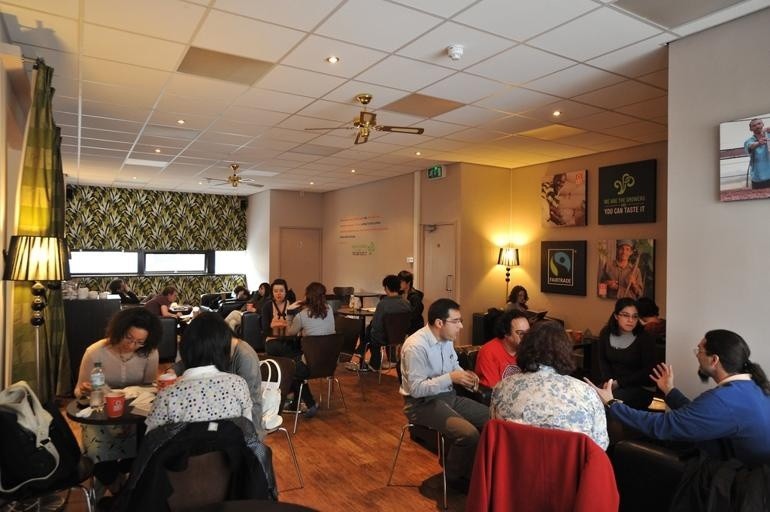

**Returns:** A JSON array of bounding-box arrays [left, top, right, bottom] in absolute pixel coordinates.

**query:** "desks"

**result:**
[[610, 431, 704, 463]]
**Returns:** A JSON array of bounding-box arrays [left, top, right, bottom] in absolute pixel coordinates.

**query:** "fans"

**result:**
[[303, 91, 425, 146], [203, 162, 266, 190]]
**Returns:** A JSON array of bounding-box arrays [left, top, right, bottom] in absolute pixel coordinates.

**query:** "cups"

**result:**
[[247, 303, 253, 312], [105, 391, 125, 417], [158, 374, 176, 389], [575, 331, 584, 343], [193, 306, 199, 317], [565, 329, 573, 339]]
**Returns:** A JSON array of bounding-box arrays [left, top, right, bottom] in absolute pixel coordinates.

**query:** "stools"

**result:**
[[36, 455, 97, 511], [387, 362, 452, 508], [259, 415, 306, 489]]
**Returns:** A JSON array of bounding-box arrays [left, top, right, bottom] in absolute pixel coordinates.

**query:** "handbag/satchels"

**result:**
[[1, 380, 54, 447], [260, 381, 284, 430], [1, 403, 82, 479]]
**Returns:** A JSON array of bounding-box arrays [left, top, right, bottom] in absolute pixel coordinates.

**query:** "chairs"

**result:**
[[155, 318, 178, 363], [333, 287, 353, 306], [201, 292, 231, 308], [234, 311, 270, 353], [163, 450, 231, 511], [318, 315, 367, 402], [292, 333, 346, 434], [465, 420, 620, 512], [200, 292, 223, 309], [361, 312, 413, 385]]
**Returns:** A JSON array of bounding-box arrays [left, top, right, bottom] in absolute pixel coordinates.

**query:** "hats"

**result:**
[[617, 240, 634, 248]]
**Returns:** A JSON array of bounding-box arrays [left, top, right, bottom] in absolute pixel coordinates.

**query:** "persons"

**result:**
[[490, 321, 609, 451], [166, 336, 264, 441], [74, 307, 163, 502], [145, 311, 253, 440], [345, 270, 424, 371], [584, 329, 769, 511], [108, 280, 178, 318], [504, 285, 537, 323], [282, 281, 335, 417], [400, 298, 490, 496], [233, 279, 293, 342], [744, 118, 770, 189], [542, 174, 586, 226], [597, 239, 645, 300], [474, 309, 529, 388], [589, 299, 665, 409]]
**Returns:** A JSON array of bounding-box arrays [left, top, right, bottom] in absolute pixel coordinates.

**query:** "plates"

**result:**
[[61, 281, 107, 300]]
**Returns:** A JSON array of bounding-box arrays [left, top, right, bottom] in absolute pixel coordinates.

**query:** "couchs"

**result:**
[[407, 344, 493, 457]]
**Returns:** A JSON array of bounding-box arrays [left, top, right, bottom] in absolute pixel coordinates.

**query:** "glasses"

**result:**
[[693, 346, 712, 354], [616, 311, 637, 318], [441, 317, 463, 325], [121, 334, 145, 348]]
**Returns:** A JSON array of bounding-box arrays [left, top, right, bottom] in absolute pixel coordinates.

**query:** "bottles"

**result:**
[[349, 295, 354, 308], [354, 297, 361, 309], [90, 362, 105, 406]]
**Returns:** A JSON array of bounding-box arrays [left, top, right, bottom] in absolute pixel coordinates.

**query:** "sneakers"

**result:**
[[303, 400, 318, 418], [353, 361, 367, 372], [382, 361, 397, 369], [367, 363, 380, 372], [283, 401, 301, 413]]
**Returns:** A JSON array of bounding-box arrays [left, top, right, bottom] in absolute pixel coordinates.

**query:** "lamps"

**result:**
[[448, 42, 464, 62], [0, 234, 70, 404], [498, 247, 519, 308]]
